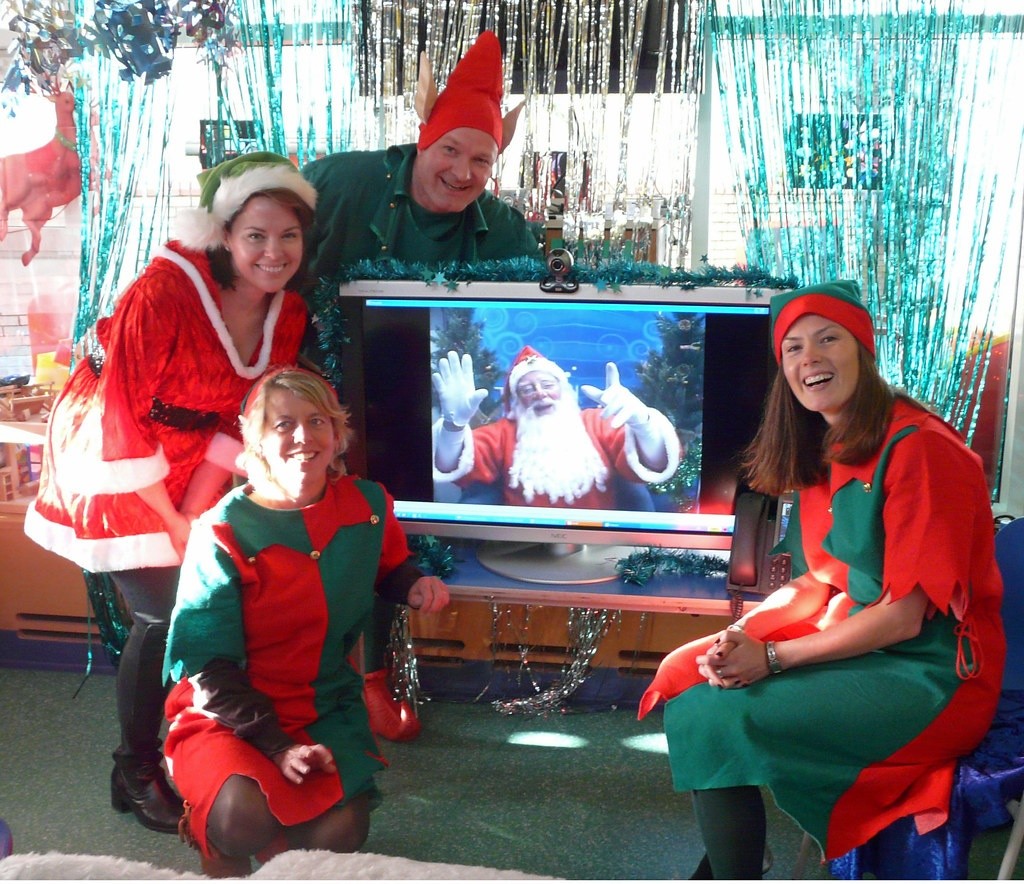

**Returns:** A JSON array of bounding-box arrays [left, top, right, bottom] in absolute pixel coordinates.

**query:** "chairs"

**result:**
[[792, 517, 1024, 880]]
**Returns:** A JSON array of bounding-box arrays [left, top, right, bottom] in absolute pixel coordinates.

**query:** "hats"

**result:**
[[238, 366, 343, 471], [414, 30, 527, 155], [769, 279, 876, 369], [174, 150, 319, 250], [503, 346, 568, 419]]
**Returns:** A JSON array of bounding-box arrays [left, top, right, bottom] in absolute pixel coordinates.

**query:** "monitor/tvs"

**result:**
[[340, 280, 792, 583]]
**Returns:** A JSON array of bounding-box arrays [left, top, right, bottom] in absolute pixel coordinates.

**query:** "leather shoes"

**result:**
[[111, 758, 185, 832]]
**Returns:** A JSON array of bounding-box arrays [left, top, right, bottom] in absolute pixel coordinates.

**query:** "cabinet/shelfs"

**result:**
[[0, 493, 767, 714]]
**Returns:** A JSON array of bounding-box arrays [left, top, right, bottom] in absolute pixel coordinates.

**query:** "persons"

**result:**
[[636, 279, 1007, 881], [25, 153, 316, 835], [433, 346, 681, 510], [159, 368, 451, 877], [302, 32, 545, 276]]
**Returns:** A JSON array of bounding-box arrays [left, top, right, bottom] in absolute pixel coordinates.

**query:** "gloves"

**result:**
[[580, 361, 649, 428], [431, 350, 488, 427]]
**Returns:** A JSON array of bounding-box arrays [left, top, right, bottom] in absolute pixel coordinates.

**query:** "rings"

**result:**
[[716, 669, 724, 677]]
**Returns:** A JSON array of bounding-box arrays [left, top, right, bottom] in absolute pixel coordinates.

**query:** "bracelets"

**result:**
[[766, 641, 781, 673]]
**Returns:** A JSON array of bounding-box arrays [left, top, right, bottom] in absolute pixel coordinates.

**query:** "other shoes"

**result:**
[[178, 802, 252, 879], [358, 668, 421, 741], [690, 841, 774, 880]]
